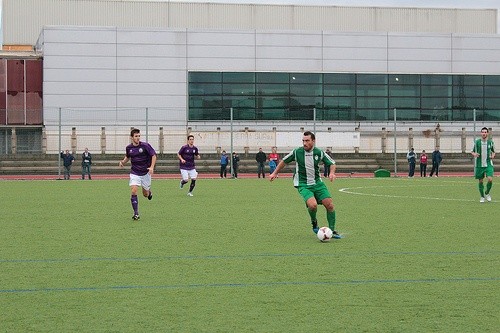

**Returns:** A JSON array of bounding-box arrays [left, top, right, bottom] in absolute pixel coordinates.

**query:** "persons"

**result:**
[[429, 146, 444, 177], [407, 148, 417, 177], [81, 148, 92, 180], [472, 127, 495, 202], [419, 150, 428, 177], [324, 149, 332, 177], [178, 136, 200, 197], [118, 129, 156, 220], [255, 147, 266, 178], [229, 151, 239, 178], [219, 151, 228, 179], [269, 131, 345, 239], [268, 149, 279, 178], [60, 149, 75, 180]]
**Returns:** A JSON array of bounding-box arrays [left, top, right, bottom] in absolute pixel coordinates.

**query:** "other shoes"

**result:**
[[180, 180, 184, 189], [134, 213, 139, 220], [148, 190, 152, 200], [313, 227, 320, 234], [331, 230, 341, 239], [187, 193, 193, 197]]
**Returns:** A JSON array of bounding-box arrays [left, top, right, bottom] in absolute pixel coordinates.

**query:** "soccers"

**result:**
[[317, 226, 332, 243]]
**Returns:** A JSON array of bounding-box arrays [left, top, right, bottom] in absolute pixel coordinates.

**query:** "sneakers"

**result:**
[[486, 194, 492, 202], [480, 197, 485, 202]]
